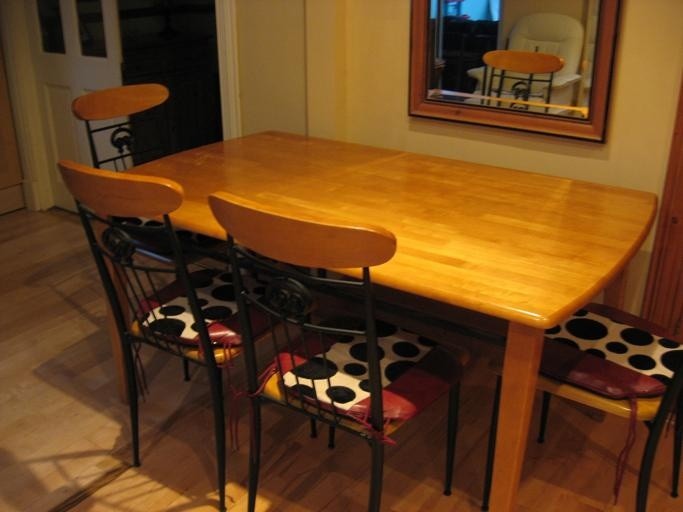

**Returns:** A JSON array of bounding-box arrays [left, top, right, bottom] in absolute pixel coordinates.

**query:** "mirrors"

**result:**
[[409, 0, 621, 145]]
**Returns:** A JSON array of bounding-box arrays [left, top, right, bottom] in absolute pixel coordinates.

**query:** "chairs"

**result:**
[[467, 13, 584, 120]]
